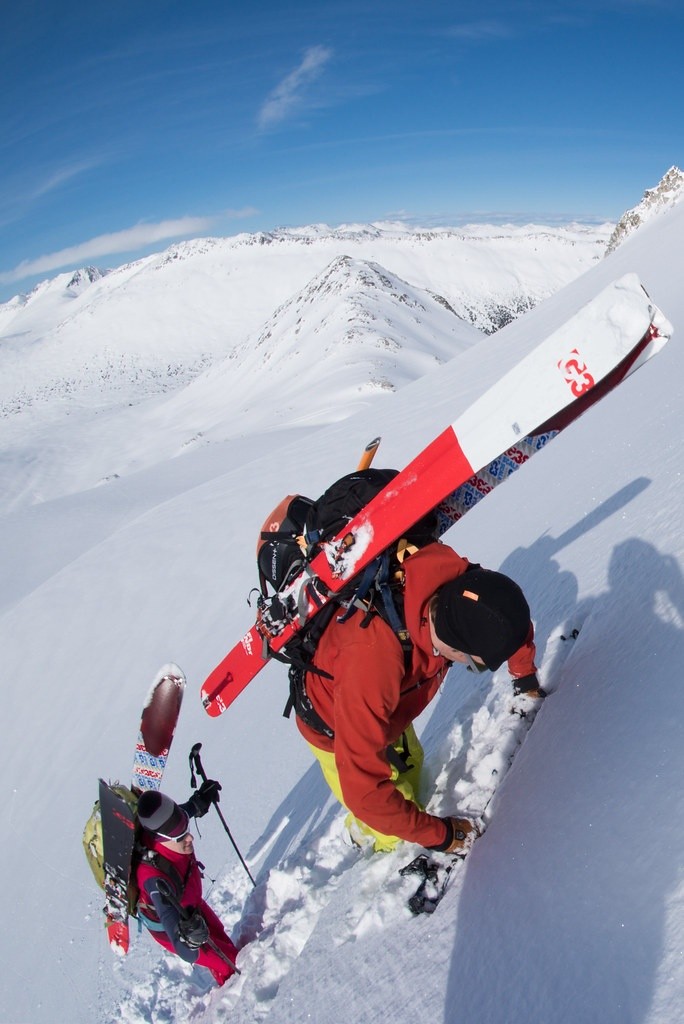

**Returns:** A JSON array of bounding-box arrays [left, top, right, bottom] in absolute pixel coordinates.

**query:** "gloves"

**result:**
[[177, 905, 209, 946], [190, 780, 222, 818]]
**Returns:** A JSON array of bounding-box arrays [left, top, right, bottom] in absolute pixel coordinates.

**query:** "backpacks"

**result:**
[[281, 469, 437, 740], [82, 782, 184, 931]]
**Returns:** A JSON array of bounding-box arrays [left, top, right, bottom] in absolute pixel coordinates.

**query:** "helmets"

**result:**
[[257, 494, 316, 591]]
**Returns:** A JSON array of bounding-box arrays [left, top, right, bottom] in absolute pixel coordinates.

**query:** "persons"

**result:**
[[132, 778, 240, 986], [296, 544, 544, 858]]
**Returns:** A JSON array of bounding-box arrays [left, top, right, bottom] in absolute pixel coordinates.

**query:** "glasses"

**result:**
[[463, 653, 489, 673], [146, 806, 191, 843]]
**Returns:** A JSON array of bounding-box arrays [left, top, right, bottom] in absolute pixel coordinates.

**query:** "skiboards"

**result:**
[[198, 269, 673, 717], [97, 661, 187, 957]]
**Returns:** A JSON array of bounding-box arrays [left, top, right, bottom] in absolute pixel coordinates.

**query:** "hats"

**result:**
[[137, 791, 188, 842], [435, 570, 530, 672]]
